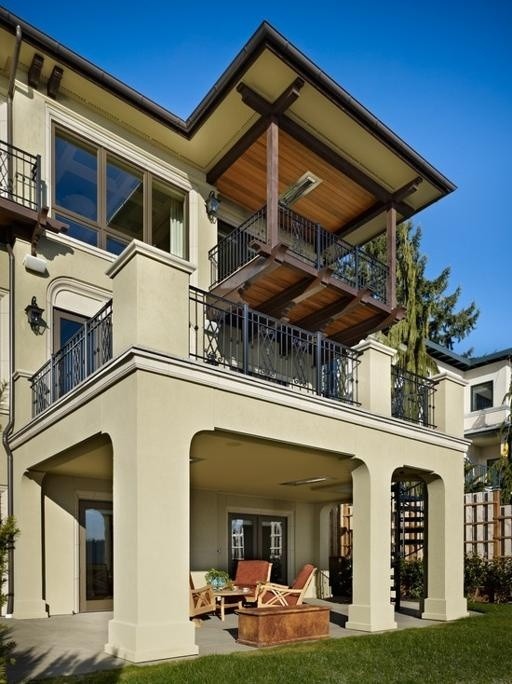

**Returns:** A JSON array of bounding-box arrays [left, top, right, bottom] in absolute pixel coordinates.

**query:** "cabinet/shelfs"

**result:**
[[235, 604, 332, 649]]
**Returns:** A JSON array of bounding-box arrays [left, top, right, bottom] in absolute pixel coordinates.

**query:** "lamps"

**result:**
[[203, 190, 221, 222], [24, 295, 45, 329]]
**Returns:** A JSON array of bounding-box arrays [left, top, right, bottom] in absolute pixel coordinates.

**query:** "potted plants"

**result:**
[[205, 567, 235, 592]]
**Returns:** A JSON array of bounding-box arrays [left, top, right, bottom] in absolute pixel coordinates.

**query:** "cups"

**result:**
[[232, 586, 250, 593]]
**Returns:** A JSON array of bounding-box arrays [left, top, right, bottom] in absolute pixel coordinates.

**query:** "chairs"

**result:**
[[229, 560, 317, 608], [189, 570, 217, 629]]
